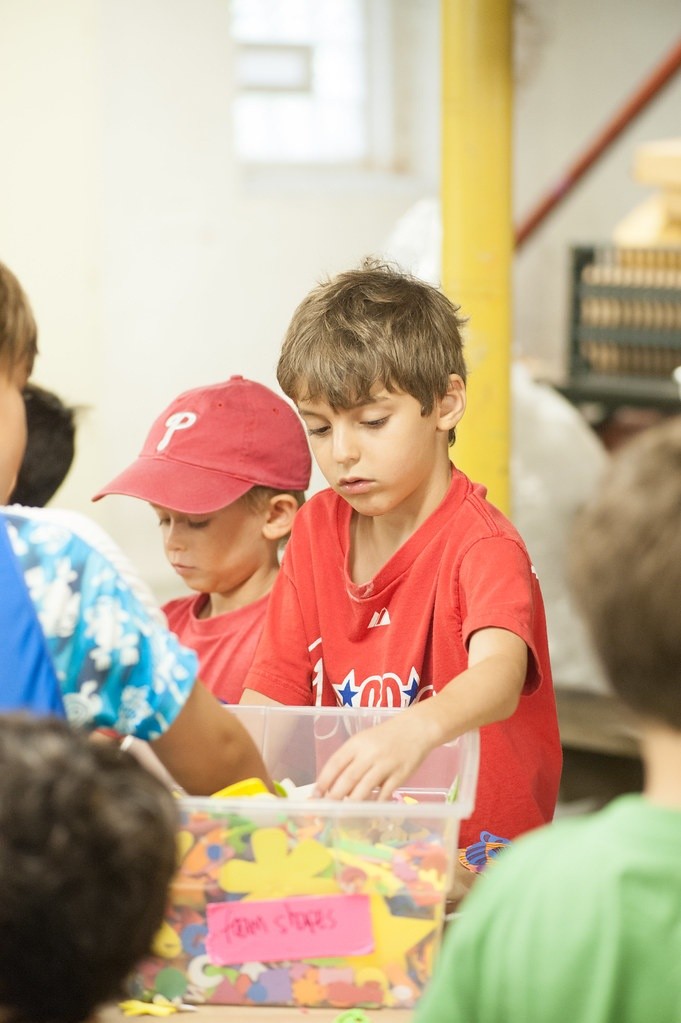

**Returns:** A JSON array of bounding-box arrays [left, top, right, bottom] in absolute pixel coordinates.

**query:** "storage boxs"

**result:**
[[126, 704, 482, 1010], [567, 247, 681, 398]]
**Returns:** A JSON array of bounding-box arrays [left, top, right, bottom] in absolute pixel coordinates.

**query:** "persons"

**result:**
[[0, 266, 279, 798], [232, 257, 560, 849], [0, 710, 180, 1023], [91, 375, 312, 704], [8, 382, 75, 507], [406, 418, 681, 1023]]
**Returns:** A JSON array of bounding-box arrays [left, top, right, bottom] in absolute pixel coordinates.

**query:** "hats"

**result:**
[[92, 376, 311, 515]]
